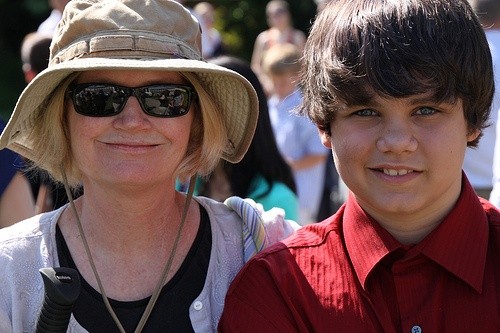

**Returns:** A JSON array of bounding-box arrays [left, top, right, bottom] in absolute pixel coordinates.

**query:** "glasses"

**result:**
[[63, 80, 198, 119]]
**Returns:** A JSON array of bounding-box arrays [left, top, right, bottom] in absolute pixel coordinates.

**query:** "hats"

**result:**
[[0, 0, 260, 164]]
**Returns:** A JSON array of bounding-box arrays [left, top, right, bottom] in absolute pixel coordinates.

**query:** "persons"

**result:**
[[0, 0, 303, 333], [74, 87, 188, 117], [174, 0, 499, 236], [215, 0, 499, 333], [0, 0, 83, 228]]
